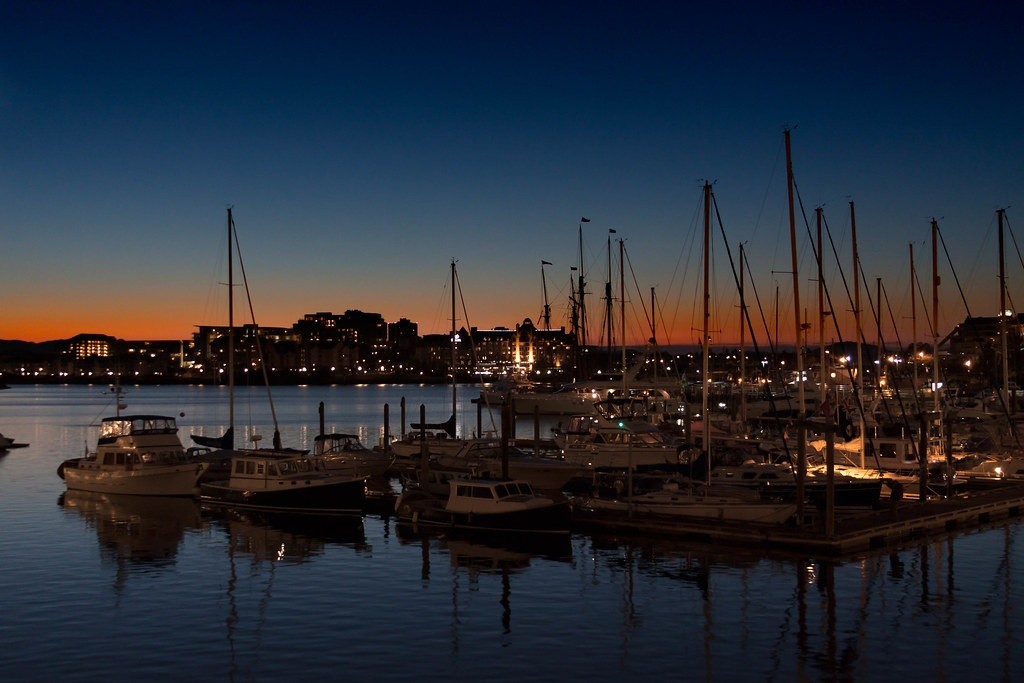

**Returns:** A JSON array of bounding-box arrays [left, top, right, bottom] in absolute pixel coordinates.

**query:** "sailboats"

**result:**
[[188, 122, 1024, 521]]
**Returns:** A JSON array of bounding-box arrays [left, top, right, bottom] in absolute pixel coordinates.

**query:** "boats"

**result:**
[[56, 414, 209, 496]]
